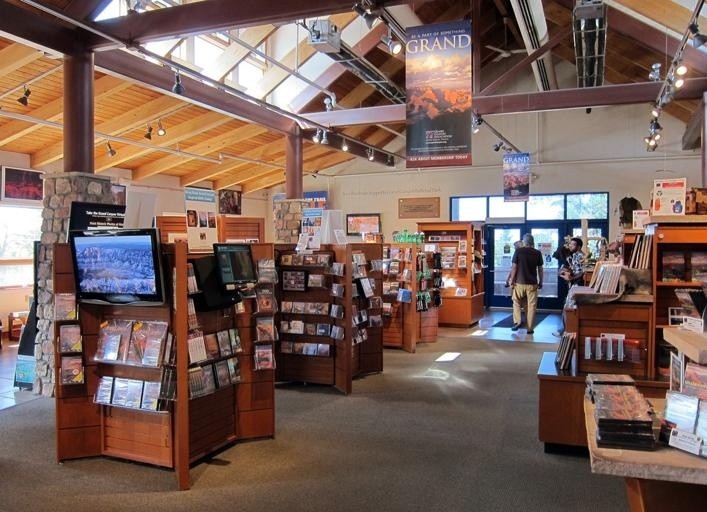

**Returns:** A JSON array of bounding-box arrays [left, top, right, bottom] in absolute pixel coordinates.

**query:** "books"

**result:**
[[280, 341, 330, 357], [281, 254, 331, 267], [281, 301, 329, 315], [589, 260, 622, 295], [238, 260, 276, 370], [330, 325, 345, 340], [330, 304, 344, 320], [663, 251, 705, 325], [173, 298, 199, 330], [330, 283, 344, 298], [283, 271, 324, 292], [622, 234, 653, 270], [172, 263, 199, 295], [383, 247, 411, 316], [188, 357, 241, 400], [425, 240, 467, 296], [93, 367, 177, 411], [93, 318, 176, 367], [331, 262, 344, 277], [280, 320, 331, 336], [167, 233, 259, 255], [56, 293, 85, 385], [351, 250, 383, 346], [584, 350, 705, 455], [554, 331, 576, 370], [187, 330, 243, 365]]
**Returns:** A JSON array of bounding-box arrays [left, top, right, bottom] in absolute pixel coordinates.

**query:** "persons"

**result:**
[[618, 193, 643, 230], [507, 241, 522, 292], [509, 233, 544, 334], [554, 234, 586, 310]]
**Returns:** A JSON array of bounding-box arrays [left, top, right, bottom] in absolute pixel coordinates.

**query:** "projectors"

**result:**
[[308, 19, 342, 55]]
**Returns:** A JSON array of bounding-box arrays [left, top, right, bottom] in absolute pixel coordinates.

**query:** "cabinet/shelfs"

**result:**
[[535, 214, 707, 459], [382, 242, 438, 354], [272, 240, 384, 395], [52, 237, 276, 491], [416, 221, 486, 327]]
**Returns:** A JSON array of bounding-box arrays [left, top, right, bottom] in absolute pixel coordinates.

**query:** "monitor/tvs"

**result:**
[[212, 242, 258, 286], [68, 228, 165, 304]]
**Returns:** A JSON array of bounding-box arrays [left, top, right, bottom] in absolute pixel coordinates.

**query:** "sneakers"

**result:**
[[512, 324, 521, 331], [527, 330, 534, 334], [553, 331, 560, 337]]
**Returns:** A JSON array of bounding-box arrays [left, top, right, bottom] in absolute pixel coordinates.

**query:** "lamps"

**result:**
[[645, 0, 707, 151], [16, 85, 31, 107], [106, 102, 190, 158], [351, 0, 521, 155], [126, 43, 408, 162]]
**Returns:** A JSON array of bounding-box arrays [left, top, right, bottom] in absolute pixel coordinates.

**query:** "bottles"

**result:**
[[188, 211, 197, 227], [394, 229, 426, 244], [363, 232, 385, 242]]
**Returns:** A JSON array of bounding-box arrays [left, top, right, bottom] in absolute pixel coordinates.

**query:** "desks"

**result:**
[[583, 393, 707, 512]]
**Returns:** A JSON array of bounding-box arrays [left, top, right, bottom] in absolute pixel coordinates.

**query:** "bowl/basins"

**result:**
[[673, 205, 682, 213]]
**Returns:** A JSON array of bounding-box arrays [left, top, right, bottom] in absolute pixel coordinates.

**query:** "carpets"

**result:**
[[492, 311, 550, 329]]
